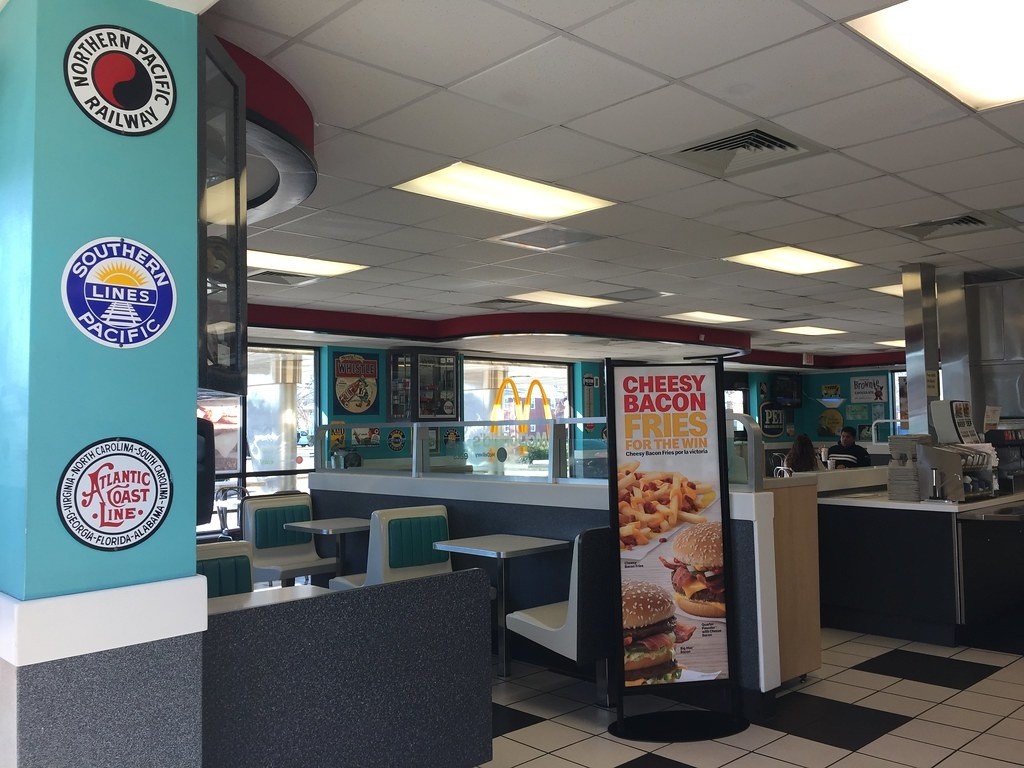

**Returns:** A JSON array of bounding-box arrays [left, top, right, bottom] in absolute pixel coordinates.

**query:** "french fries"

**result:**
[[617, 459, 716, 550]]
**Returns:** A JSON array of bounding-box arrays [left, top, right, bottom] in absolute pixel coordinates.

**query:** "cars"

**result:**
[[297, 430, 314, 447]]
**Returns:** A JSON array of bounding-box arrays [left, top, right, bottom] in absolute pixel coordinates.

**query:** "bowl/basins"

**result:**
[[887, 434, 932, 501]]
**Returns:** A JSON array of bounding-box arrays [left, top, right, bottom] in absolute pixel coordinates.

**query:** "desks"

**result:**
[[433, 533, 569, 679], [284, 516, 369, 577]]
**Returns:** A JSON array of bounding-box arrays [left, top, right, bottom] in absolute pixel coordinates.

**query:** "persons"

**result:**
[[821, 427, 873, 470], [782, 434, 828, 481]]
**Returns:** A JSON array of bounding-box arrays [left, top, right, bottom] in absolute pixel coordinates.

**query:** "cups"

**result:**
[[822, 448, 828, 462], [827, 460, 835, 471]]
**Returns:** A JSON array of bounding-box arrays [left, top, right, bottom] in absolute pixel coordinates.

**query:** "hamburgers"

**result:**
[[660, 522, 728, 618], [621, 579, 697, 686]]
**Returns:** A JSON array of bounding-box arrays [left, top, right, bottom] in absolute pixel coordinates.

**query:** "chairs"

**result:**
[[194, 541, 254, 598], [505, 525, 618, 708], [241, 492, 337, 588], [328, 504, 450, 596]]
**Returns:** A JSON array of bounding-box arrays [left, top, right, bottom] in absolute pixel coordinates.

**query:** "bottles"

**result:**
[[344, 448, 361, 469], [331, 451, 344, 469]]
